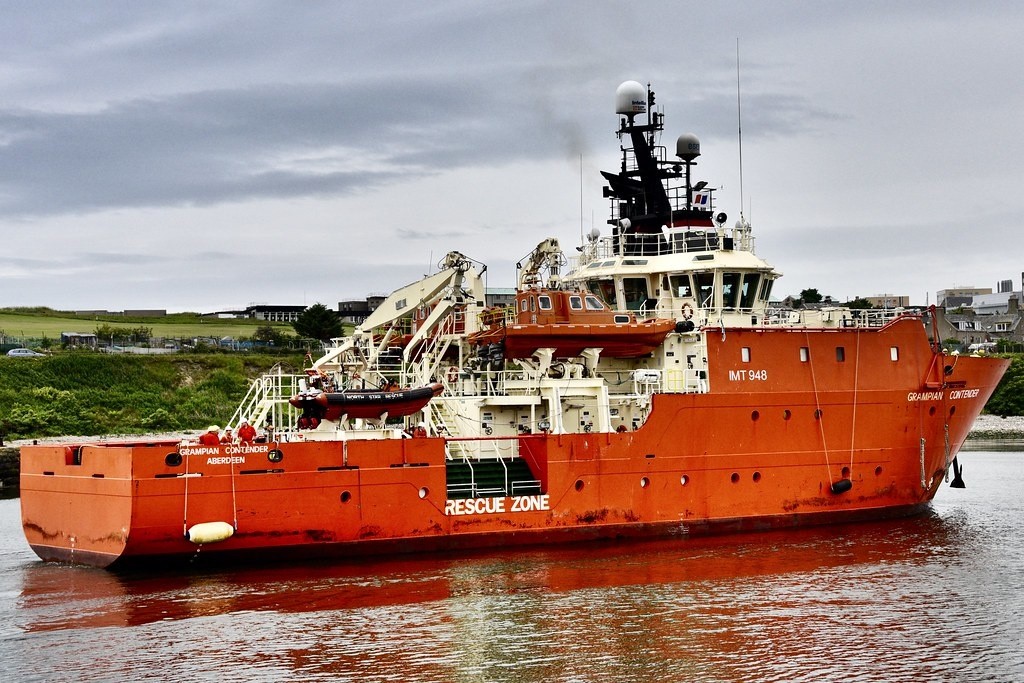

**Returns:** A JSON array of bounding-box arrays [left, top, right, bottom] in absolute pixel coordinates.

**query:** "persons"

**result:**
[[199, 425, 233, 445], [237, 418, 257, 444]]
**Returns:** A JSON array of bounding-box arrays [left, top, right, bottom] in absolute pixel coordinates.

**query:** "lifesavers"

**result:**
[[482, 309, 494, 324], [681, 302, 693, 319], [491, 306, 503, 322], [448, 366, 458, 381]]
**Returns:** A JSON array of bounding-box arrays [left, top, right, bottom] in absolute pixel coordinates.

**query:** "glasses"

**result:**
[[943, 350, 948, 353]]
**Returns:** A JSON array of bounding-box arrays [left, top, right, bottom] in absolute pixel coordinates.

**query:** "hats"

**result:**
[[943, 348, 948, 352]]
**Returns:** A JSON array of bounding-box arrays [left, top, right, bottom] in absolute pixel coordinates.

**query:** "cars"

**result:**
[[6, 349, 47, 357]]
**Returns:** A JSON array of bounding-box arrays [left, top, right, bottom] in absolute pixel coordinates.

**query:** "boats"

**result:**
[[466, 237, 680, 361], [15, 80, 1016, 573], [287, 382, 445, 430]]
